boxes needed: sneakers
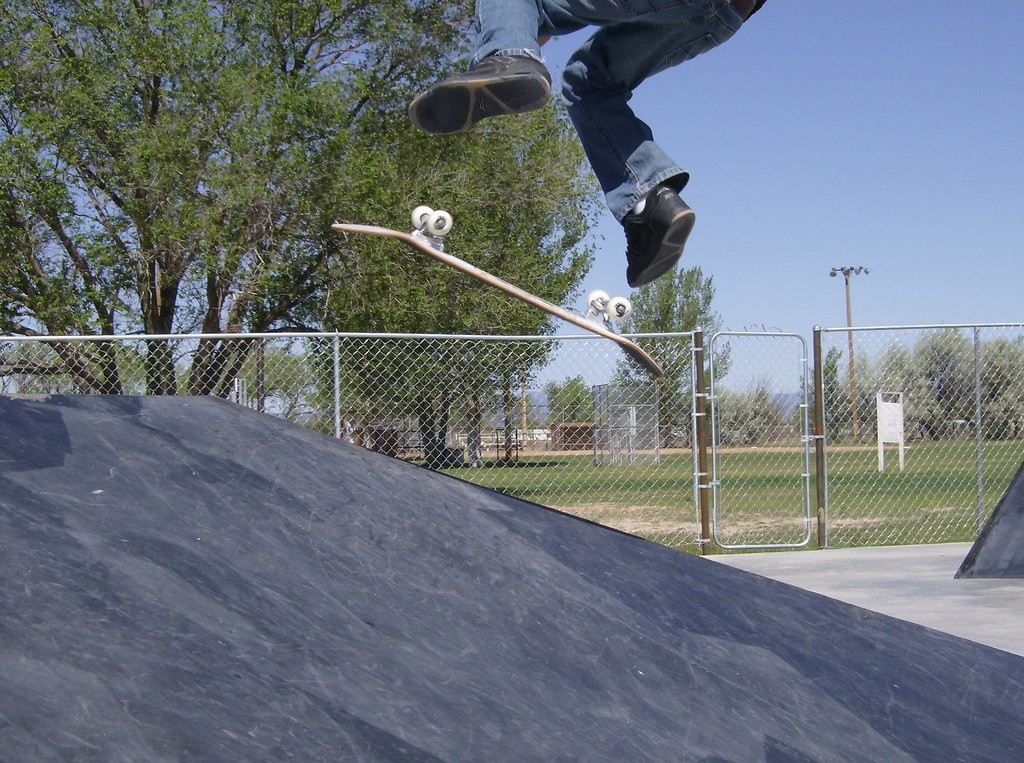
[622,185,696,287]
[408,54,552,135]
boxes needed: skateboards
[325,203,668,377]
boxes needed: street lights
[829,266,871,440]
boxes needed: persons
[408,0,767,287]
